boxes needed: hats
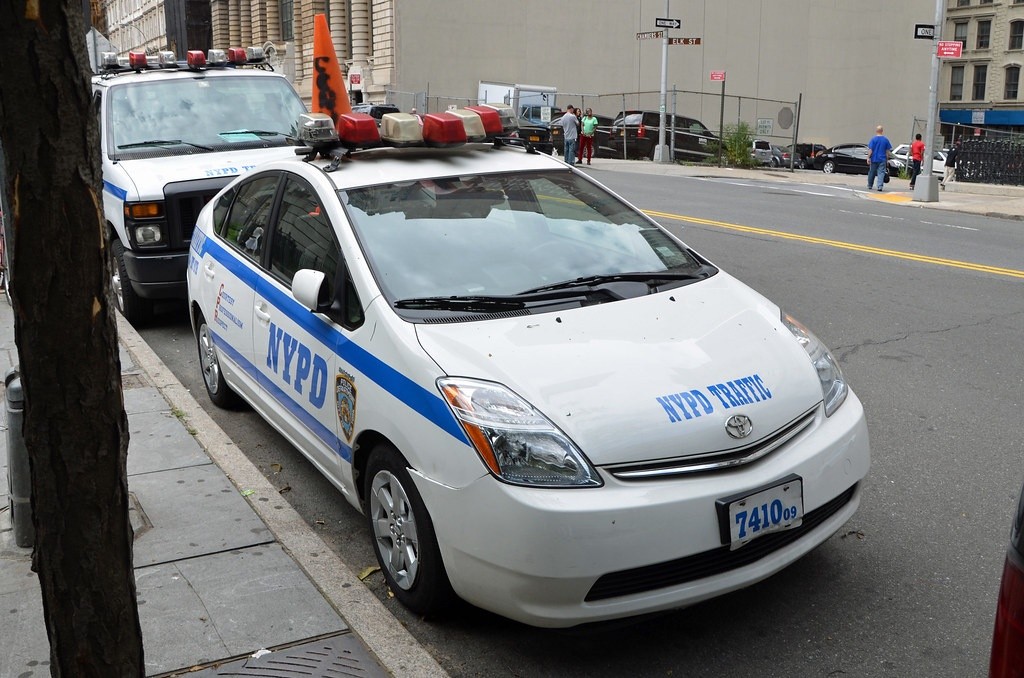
[566,104,574,109]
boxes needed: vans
[91,47,309,329]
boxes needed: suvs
[747,140,826,168]
[609,110,728,161]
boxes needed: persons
[866,124,892,191]
[561,103,599,166]
[940,141,961,190]
[909,133,926,187]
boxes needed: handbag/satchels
[884,173,889,183]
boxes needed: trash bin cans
[3,365,35,549]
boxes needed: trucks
[477,81,562,127]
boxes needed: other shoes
[576,161,582,164]
[910,185,914,190]
[877,189,882,191]
[587,161,590,165]
[940,184,945,192]
[869,187,872,189]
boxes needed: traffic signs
[655,18,681,30]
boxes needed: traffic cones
[312,13,353,135]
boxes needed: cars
[187,104,871,629]
[892,144,984,179]
[813,143,922,177]
[502,113,614,157]
